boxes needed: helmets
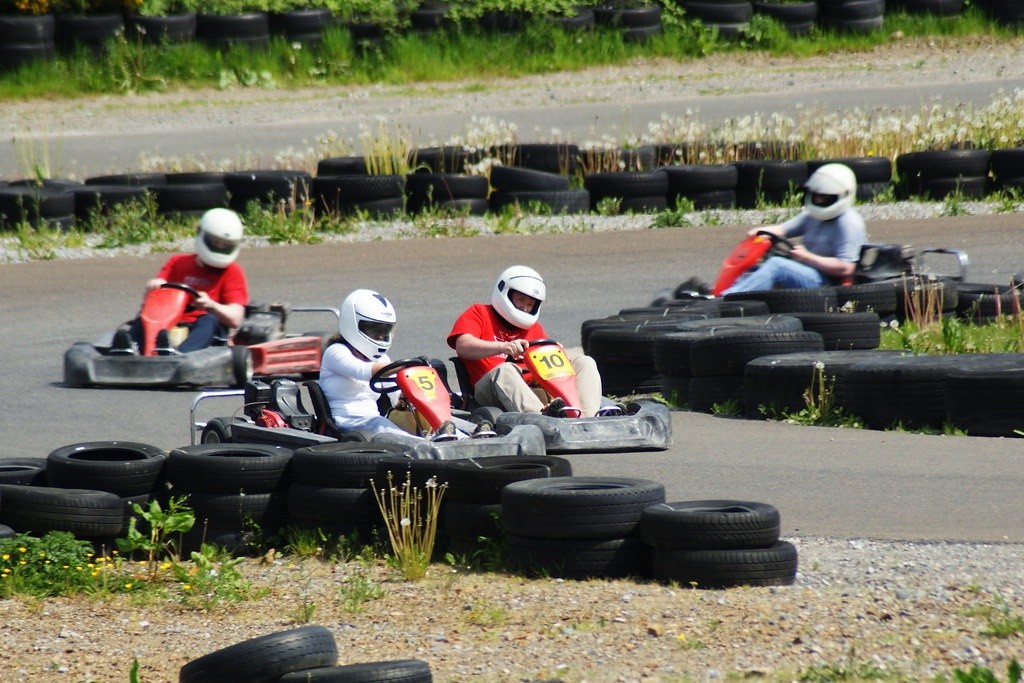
[804,163,857,221]
[195,208,244,268]
[338,289,397,362]
[491,265,547,329]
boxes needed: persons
[674,163,869,301]
[112,207,250,359]
[446,265,602,418]
[318,288,495,442]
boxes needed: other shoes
[112,325,132,348]
[155,329,171,355]
[467,419,493,440]
[540,397,566,418]
[427,419,456,441]
